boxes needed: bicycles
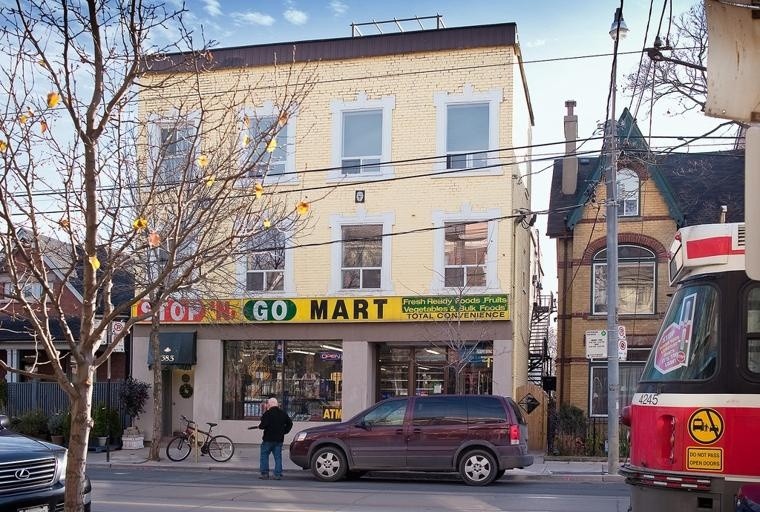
[167,414,235,461]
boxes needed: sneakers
[260,475,270,479]
[271,474,281,478]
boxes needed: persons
[257,397,294,479]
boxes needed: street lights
[599,10,631,472]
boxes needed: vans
[289,393,534,485]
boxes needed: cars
[0,414,93,512]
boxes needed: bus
[618,223,760,512]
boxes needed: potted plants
[14,399,118,449]
